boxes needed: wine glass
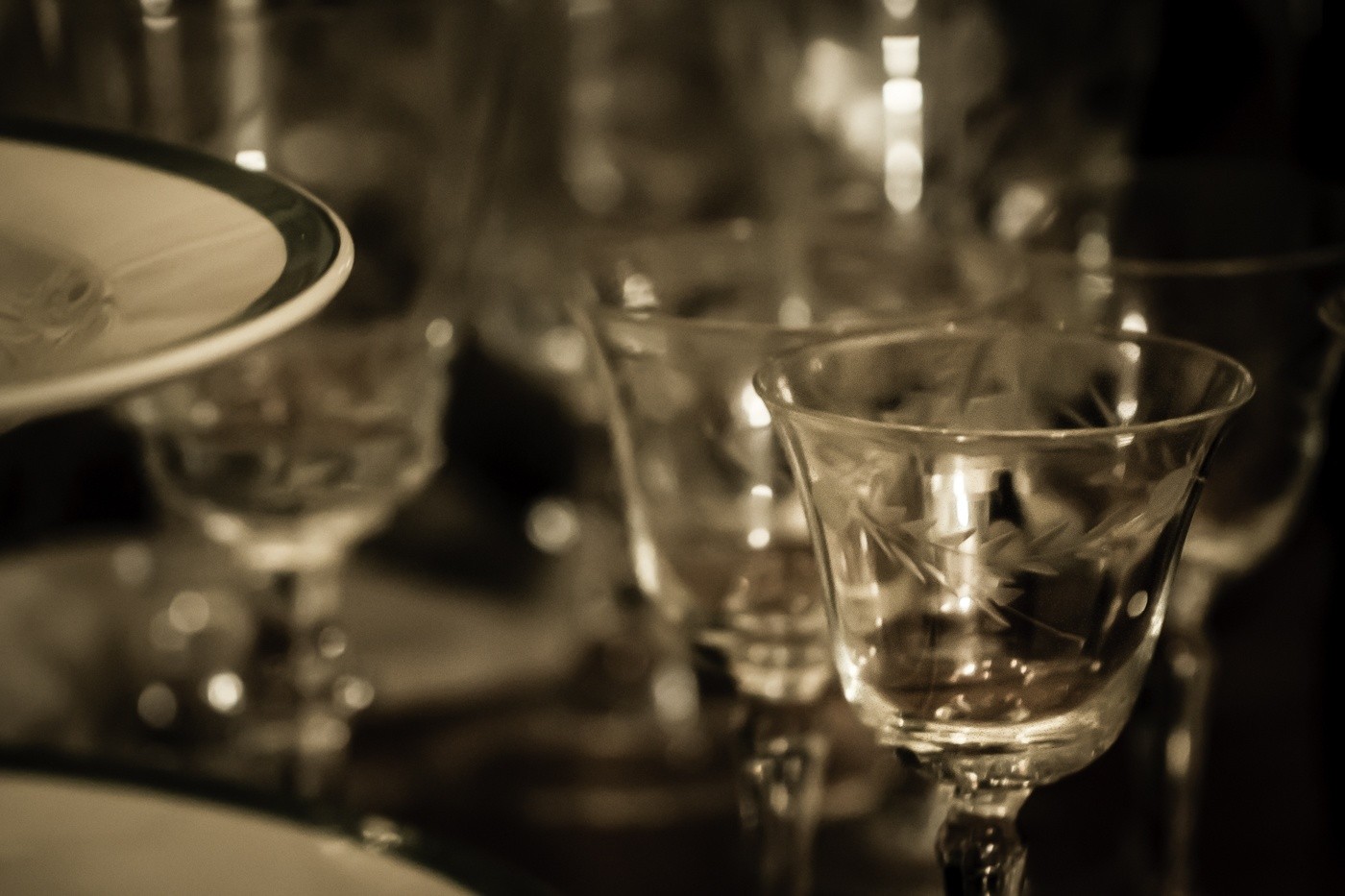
[560,164,1344,896]
[130,208,459,862]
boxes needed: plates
[0,118,357,433]
[0,746,546,896]
